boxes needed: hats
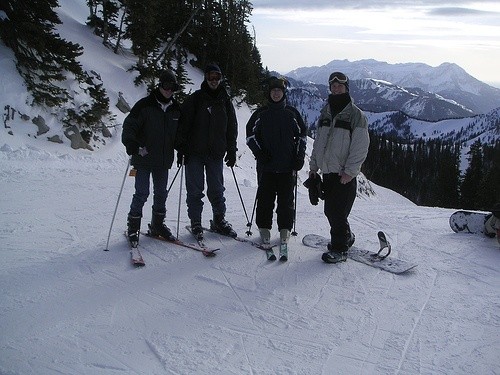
[205,64,221,72]
[269,79,286,104]
[159,69,176,83]
[329,72,350,92]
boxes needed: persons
[246,76,307,243]
[309,72,370,263]
[484,201,500,244]
[121,69,182,246]
[177,64,238,239]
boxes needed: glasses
[161,82,178,91]
[205,71,222,81]
[329,71,347,84]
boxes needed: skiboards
[122,229,221,267]
[184,224,279,257]
[259,230,288,262]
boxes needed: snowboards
[301,233,418,274]
[448,210,496,238]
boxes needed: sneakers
[191,221,203,241]
[280,229,291,245]
[210,219,237,238]
[259,228,270,244]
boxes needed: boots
[127,213,139,242]
[150,211,172,237]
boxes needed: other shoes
[322,250,347,263]
[327,234,355,252]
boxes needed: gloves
[294,155,304,171]
[303,173,326,205]
[256,150,272,162]
[225,148,237,167]
[176,144,191,165]
[130,154,147,170]
